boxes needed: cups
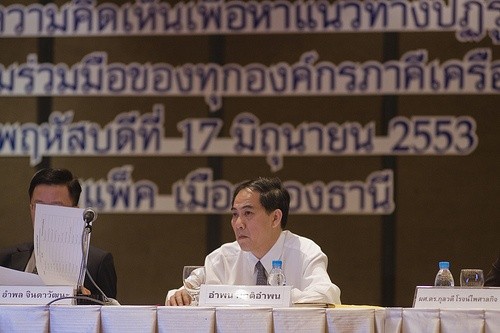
[460,269,484,286]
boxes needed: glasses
[29,203,37,210]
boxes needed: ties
[255,261,268,285]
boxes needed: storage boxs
[0,303,500,333]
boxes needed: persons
[164,177,342,307]
[483,256,500,288]
[0,167,117,305]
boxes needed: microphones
[83,207,98,224]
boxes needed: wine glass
[182,265,206,306]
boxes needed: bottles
[435,262,454,286]
[267,260,286,286]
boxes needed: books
[292,302,335,308]
